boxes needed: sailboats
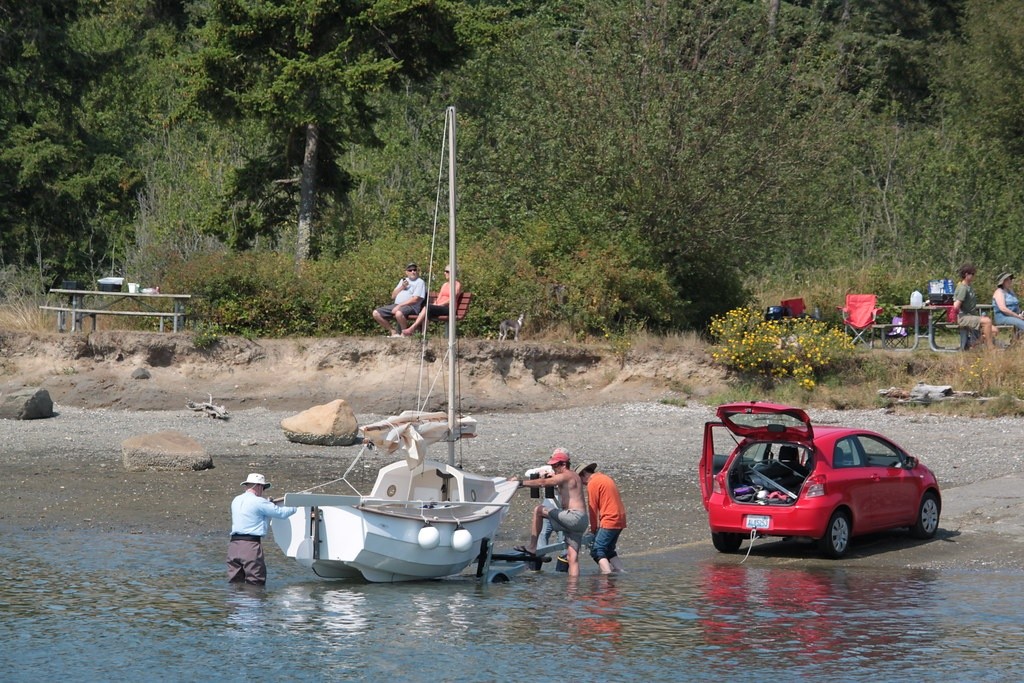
[266,108,522,584]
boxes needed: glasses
[970,272,975,275]
[405,269,417,272]
[444,270,449,273]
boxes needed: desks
[896,305,994,353]
[49,288,192,332]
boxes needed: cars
[696,402,943,559]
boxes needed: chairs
[834,446,844,466]
[835,294,882,349]
[778,446,800,464]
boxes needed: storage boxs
[98,277,124,292]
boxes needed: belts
[228,534,262,544]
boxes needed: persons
[227,474,297,586]
[993,272,1024,330]
[511,452,588,575]
[573,463,626,572]
[372,263,425,337]
[525,448,570,549]
[954,263,999,352]
[403,263,461,337]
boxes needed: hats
[404,260,418,267]
[995,272,1013,288]
[549,447,569,458]
[546,453,569,466]
[572,462,598,480]
[239,473,270,490]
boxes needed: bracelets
[519,480,523,486]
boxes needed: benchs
[387,291,471,338]
[948,324,1014,347]
[868,321,948,350]
[38,305,185,330]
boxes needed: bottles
[910,290,922,306]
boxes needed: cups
[128,283,135,293]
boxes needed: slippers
[513,545,535,556]
